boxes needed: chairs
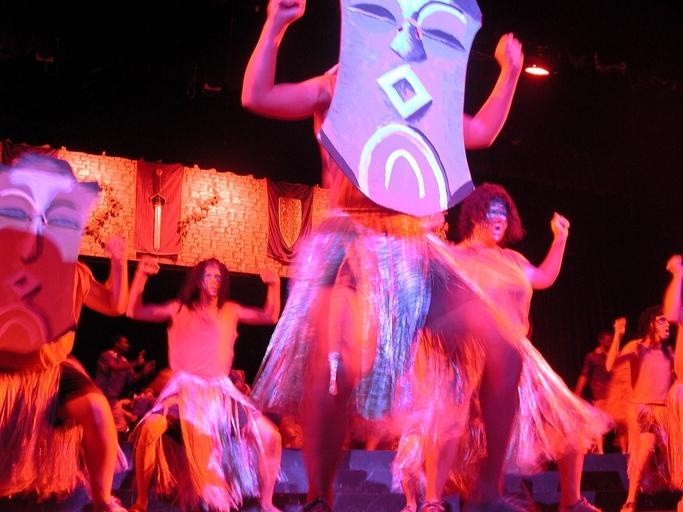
[566,495,606,512]
[501,487,542,512]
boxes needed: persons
[0,228,135,512]
[116,250,286,512]
[575,328,628,453]
[658,257,683,512]
[239,0,526,512]
[434,182,599,511]
[89,335,157,407]
[604,304,677,512]
